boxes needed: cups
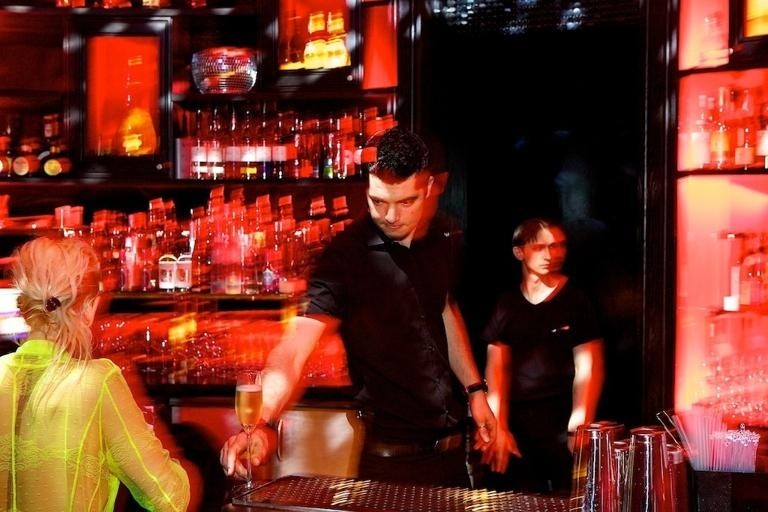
[704,349,767,429]
[425,3,594,38]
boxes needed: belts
[369,433,464,458]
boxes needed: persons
[0,234,203,512]
[482,211,607,497]
[217,127,497,490]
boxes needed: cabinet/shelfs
[644,1,768,512]
[1,1,418,427]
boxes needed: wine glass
[235,369,266,494]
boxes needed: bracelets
[465,379,490,392]
[567,430,578,438]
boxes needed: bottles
[283,11,351,74]
[697,86,767,171]
[718,230,767,311]
[1,107,73,178]
[186,107,397,182]
[88,193,352,292]
[91,296,235,371]
[567,422,689,511]
